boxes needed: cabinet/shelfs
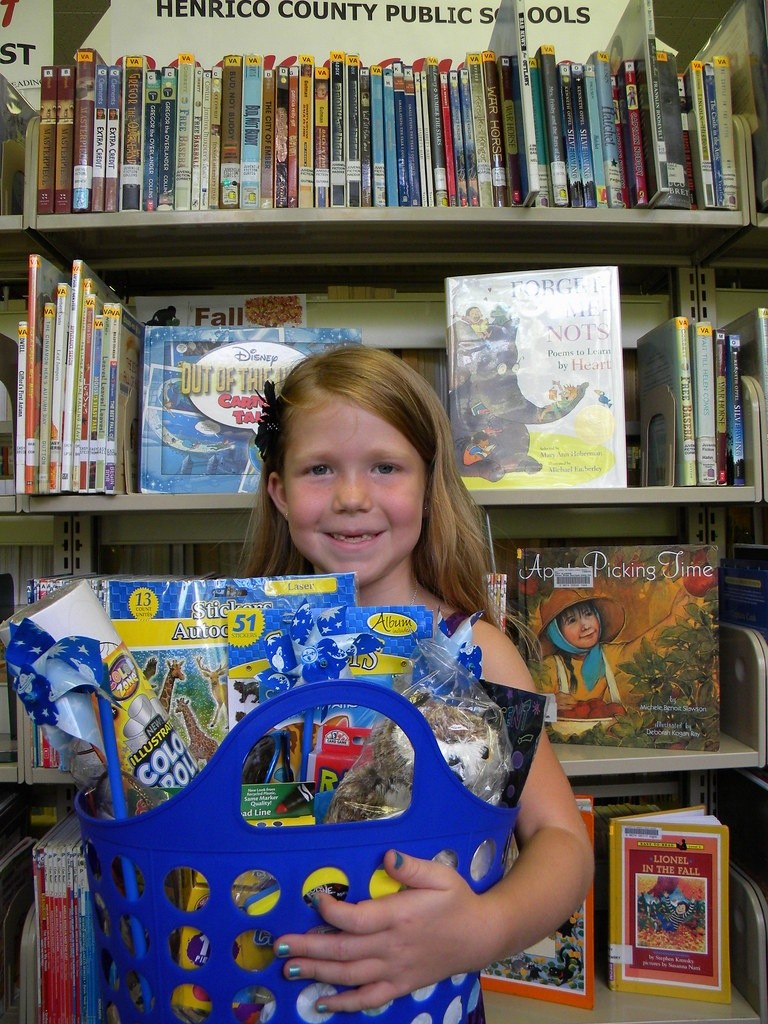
[0,0,768,1024]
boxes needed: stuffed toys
[318,686,507,828]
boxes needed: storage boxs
[137,321,365,494]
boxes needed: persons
[231,344,590,1013]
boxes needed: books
[0,37,768,1023]
